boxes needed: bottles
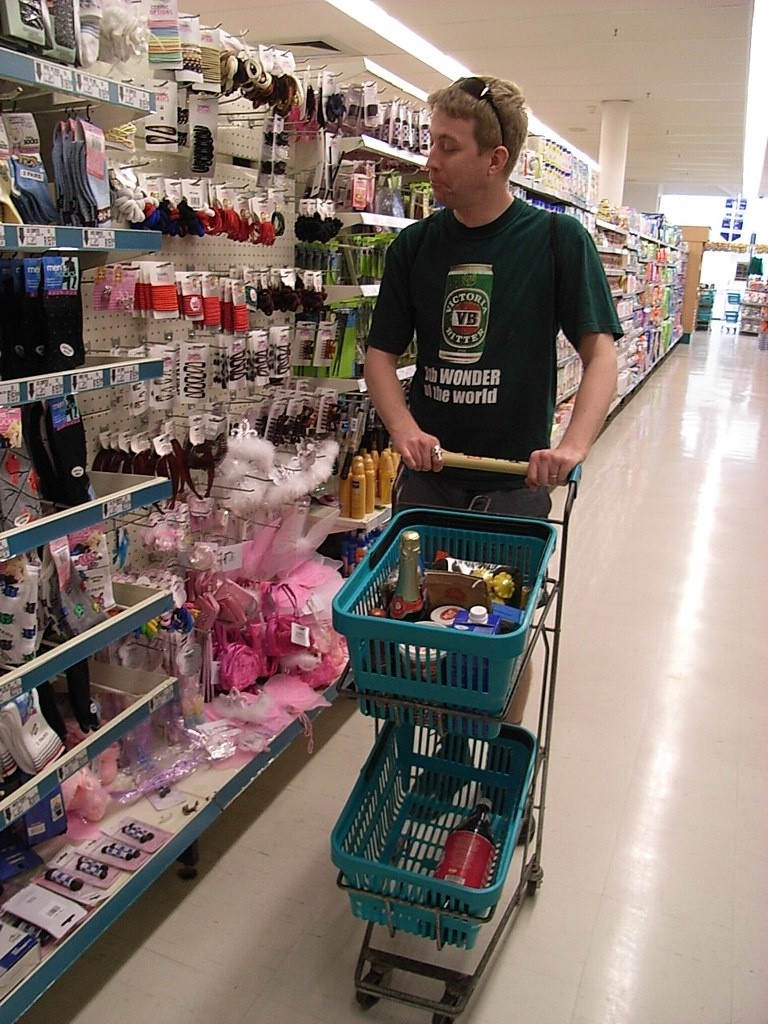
[388,531,427,682]
[420,798,495,939]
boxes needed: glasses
[450,77,506,147]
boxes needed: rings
[433,445,442,461]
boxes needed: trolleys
[719,288,742,335]
[695,283,718,333]
[329,442,584,1024]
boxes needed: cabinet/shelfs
[739,274,768,351]
[0,0,691,1024]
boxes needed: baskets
[699,293,712,306]
[330,721,537,950]
[330,508,558,741]
[725,311,738,323]
[697,310,711,322]
[728,293,740,304]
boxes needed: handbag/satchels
[185,564,346,692]
[749,256,763,275]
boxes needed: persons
[364,73,620,842]
